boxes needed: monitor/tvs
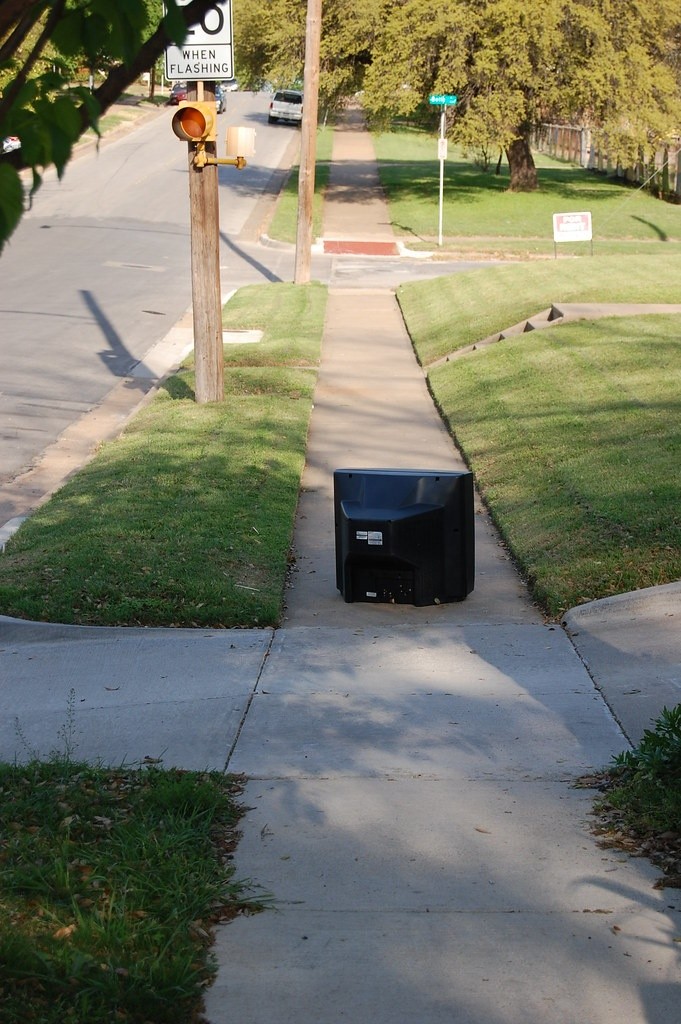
[335,468,475,607]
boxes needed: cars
[213,86,226,114]
[171,81,188,104]
[268,89,304,126]
